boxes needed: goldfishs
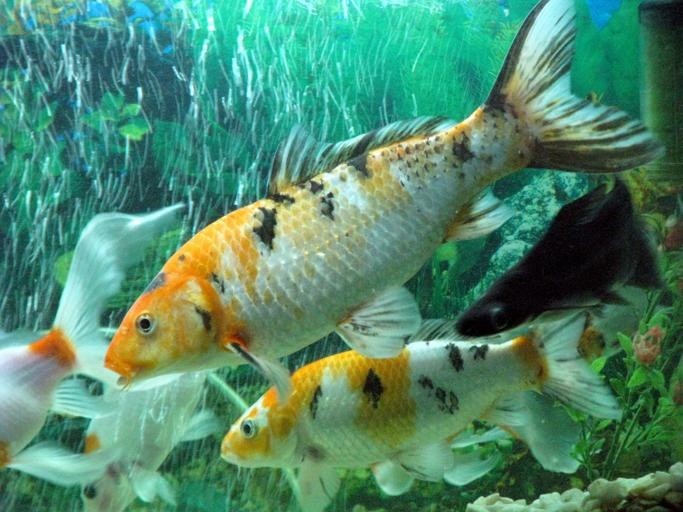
[0,201,189,488]
[80,370,228,511]
